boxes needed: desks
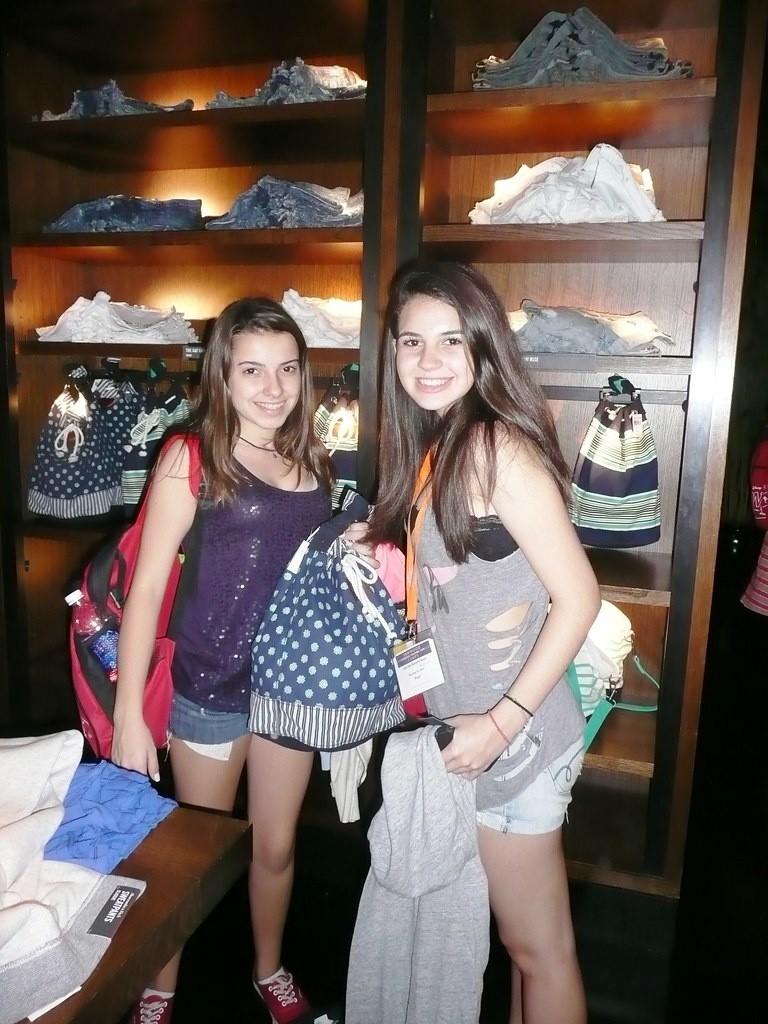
[0,805,253,1024]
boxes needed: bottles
[64,588,120,689]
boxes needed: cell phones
[434,724,500,772]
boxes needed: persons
[361,257,600,1024]
[110,296,332,1024]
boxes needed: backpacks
[70,433,202,759]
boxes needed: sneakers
[253,968,315,1024]
[128,994,175,1024]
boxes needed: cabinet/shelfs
[0,0,768,903]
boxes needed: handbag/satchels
[247,490,415,751]
[548,598,660,752]
[26,356,191,523]
[313,363,362,514]
[565,375,661,548]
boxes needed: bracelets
[487,710,512,745]
[503,693,534,717]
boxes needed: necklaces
[235,434,278,459]
